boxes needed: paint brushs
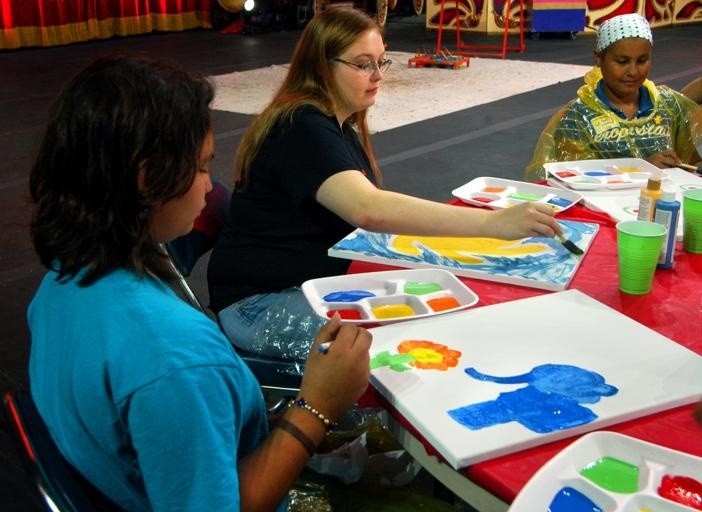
[677,164,701,173]
[557,234,583,254]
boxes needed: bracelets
[287,399,334,437]
[276,417,316,458]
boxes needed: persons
[26,50,375,512]
[207,8,562,361]
[521,11,702,180]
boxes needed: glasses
[329,55,393,75]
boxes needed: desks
[347,185,701,512]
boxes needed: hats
[596,12,654,54]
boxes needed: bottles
[638,179,662,222]
[653,192,680,269]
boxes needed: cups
[614,218,666,296]
[682,189,701,253]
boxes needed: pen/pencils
[318,340,335,352]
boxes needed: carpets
[202,52,592,135]
